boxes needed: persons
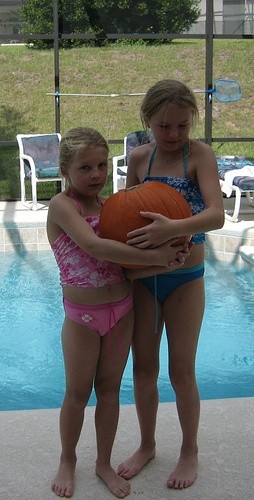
[44,123,197,500]
[117,79,226,491]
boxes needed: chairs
[214,154,254,224]
[15,132,67,213]
[111,129,155,195]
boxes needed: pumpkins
[100,180,194,269]
